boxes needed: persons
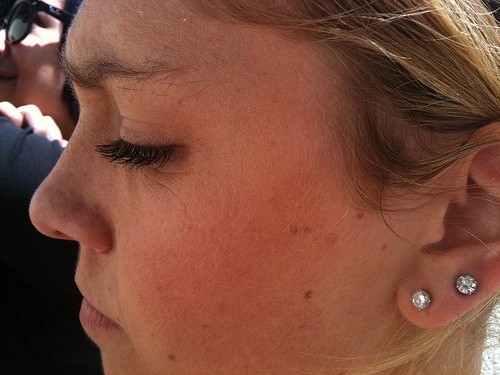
[0,0,82,147]
[29,0,500,375]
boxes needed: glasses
[0,0,74,45]
